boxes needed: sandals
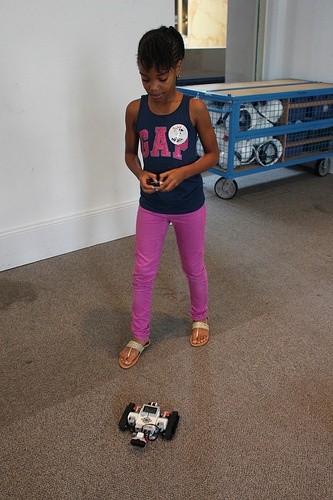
[190,316,209,346]
[118,339,150,368]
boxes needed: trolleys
[175,78,333,201]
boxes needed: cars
[118,401,180,448]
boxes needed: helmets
[189,99,285,169]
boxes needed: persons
[118,25,219,370]
